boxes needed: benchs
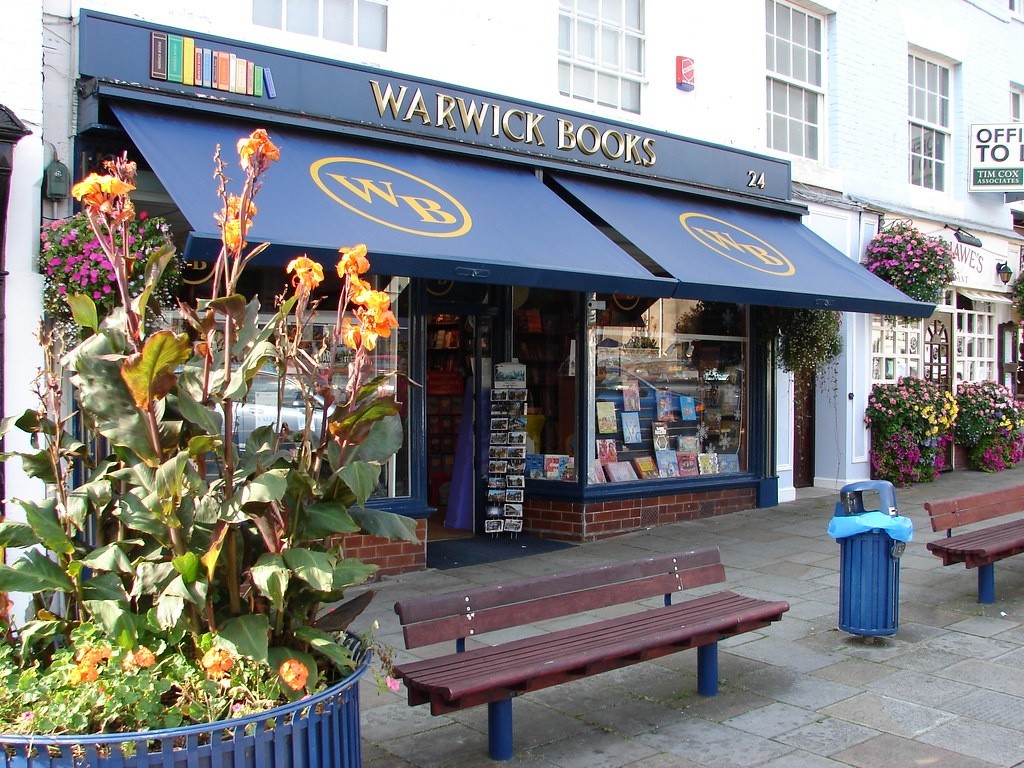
[391,547,790,761]
[924,483,1024,604]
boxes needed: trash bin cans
[836,479,907,639]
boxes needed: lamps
[944,224,982,247]
[996,261,1013,284]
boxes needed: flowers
[0,130,417,756]
[861,375,1024,487]
[862,220,958,331]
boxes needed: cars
[175,370,336,455]
[596,364,706,447]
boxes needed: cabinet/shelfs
[427,301,559,454]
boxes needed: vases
[0,630,370,768]
